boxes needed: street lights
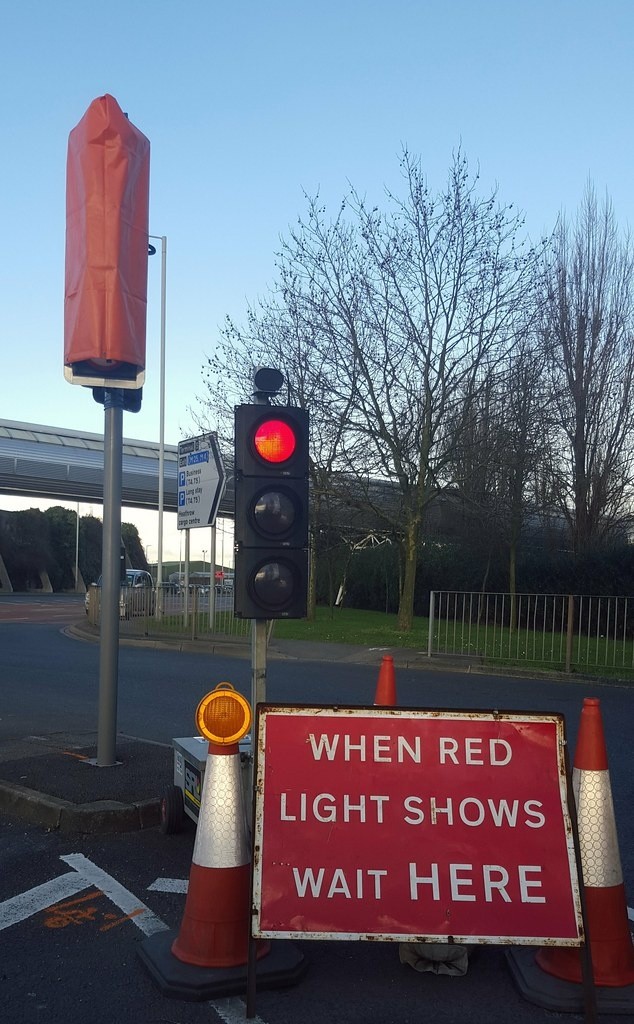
[202,550,208,573]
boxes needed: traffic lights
[232,403,314,623]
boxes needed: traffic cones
[128,687,323,1004]
[502,696,634,1016]
[376,656,399,707]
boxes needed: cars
[85,569,155,618]
[160,582,232,594]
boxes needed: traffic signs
[177,432,228,530]
